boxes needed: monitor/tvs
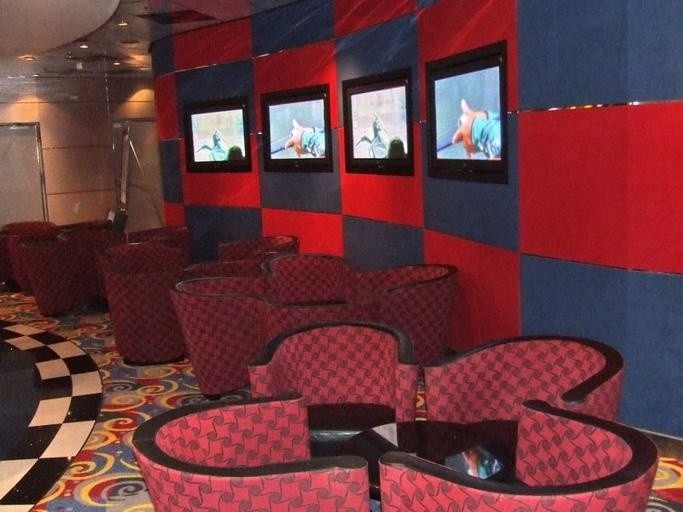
[266,92,330,165]
[346,79,413,166]
[188,104,248,165]
[430,55,506,171]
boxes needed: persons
[371,116,387,142]
[285,118,326,160]
[452,97,501,160]
[225,145,245,161]
[386,139,405,158]
[210,129,223,149]
